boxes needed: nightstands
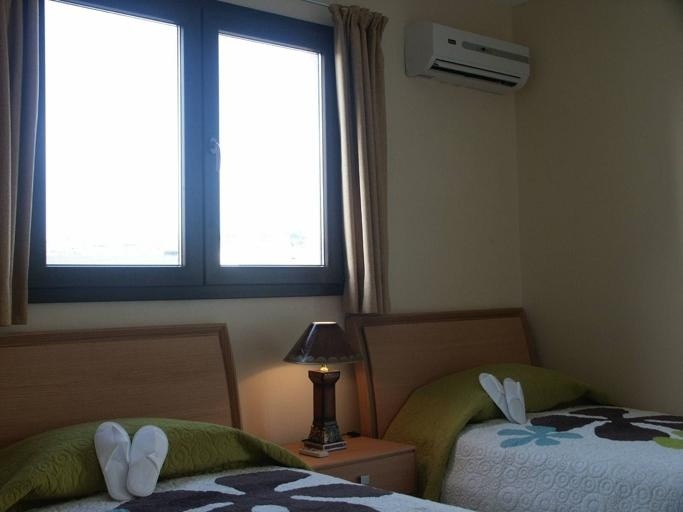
[279,433,418,495]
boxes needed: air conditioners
[404,22,530,96]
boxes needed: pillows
[436,361,574,424]
[7,418,255,507]
[444,363,582,421]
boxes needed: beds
[1,321,480,512]
[344,307,683,512]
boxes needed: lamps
[281,321,365,451]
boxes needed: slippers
[94,421,131,500]
[126,424,170,494]
[503,375,525,424]
[479,373,510,420]
